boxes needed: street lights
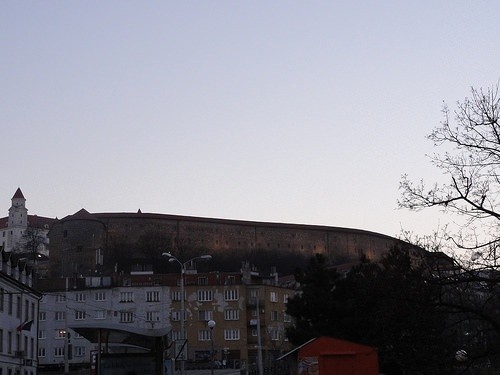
[161,251,212,375]
[208,320,216,375]
